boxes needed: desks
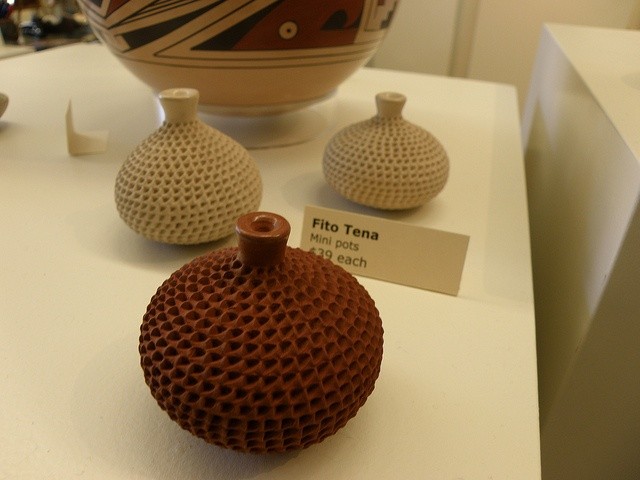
[2,44,544,480]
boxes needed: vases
[323,92,448,206]
[138,211,384,455]
[78,1,398,151]
[114,87,263,245]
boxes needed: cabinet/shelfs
[521,22,636,472]
